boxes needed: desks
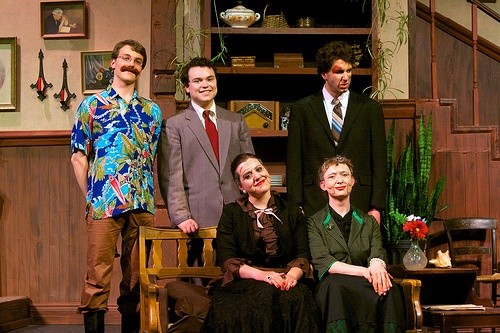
[388,267,479,305]
[421,304,500,333]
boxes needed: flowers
[388,207,428,244]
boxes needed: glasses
[119,55,143,66]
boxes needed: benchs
[139,225,219,333]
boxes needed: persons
[285,41,387,224]
[306,155,405,333]
[70,41,162,333]
[156,57,256,287]
[201,152,319,333]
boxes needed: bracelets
[369,257,386,269]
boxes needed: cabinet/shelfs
[200,0,379,194]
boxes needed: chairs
[443,217,500,307]
[392,277,423,333]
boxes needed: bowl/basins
[220,5,261,29]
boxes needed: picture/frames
[80,51,114,95]
[40,0,88,40]
[0,36,18,112]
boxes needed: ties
[204,109,219,162]
[331,99,344,143]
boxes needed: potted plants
[383,109,452,264]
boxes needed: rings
[268,276,271,280]
[281,276,285,279]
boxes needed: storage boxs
[230,56,256,67]
[230,99,280,131]
[273,53,304,69]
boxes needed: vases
[402,243,427,271]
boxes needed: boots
[83,311,105,333]
[121,313,140,333]
